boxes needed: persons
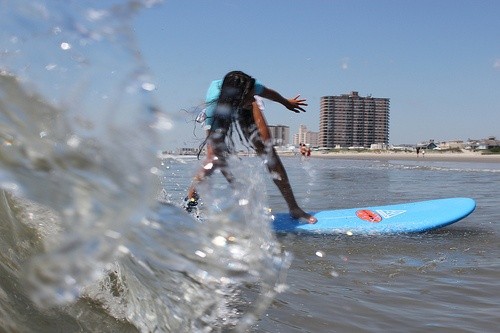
[185,70,319,224]
[416,144,421,159]
[299,143,311,157]
[422,148,425,158]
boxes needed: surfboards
[266,196,476,236]
